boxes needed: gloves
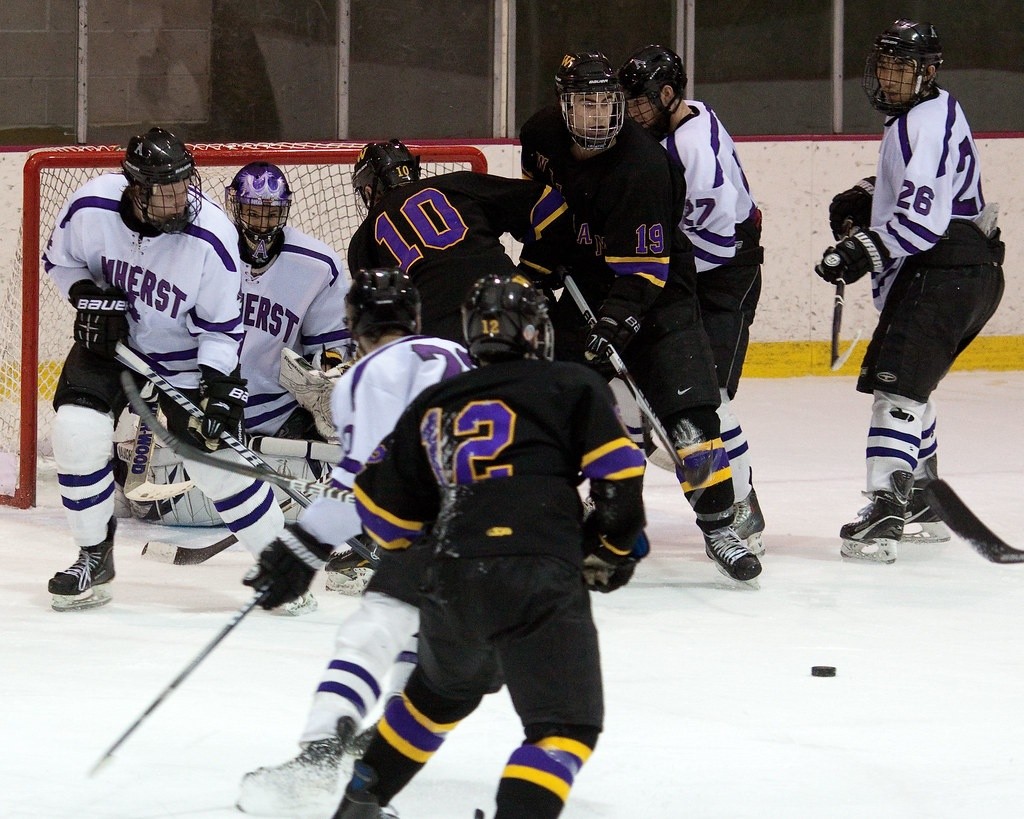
[815,232,887,286]
[68,279,132,361]
[188,374,248,453]
[244,523,334,610]
[582,528,635,592]
[579,298,641,380]
[828,176,877,240]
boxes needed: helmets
[352,139,420,222]
[121,126,201,233]
[863,20,942,114]
[225,162,292,246]
[556,51,625,150]
[618,44,688,131]
[343,266,423,339]
[459,271,557,364]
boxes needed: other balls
[812,665,836,677]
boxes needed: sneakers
[326,539,379,594]
[333,762,399,819]
[254,581,317,616]
[48,517,117,611]
[696,518,762,590]
[240,740,344,805]
[839,470,915,564]
[863,453,951,543]
[583,495,597,523]
[706,466,765,562]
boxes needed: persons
[236,269,482,819]
[328,277,649,819]
[816,19,1005,561]
[48,43,769,615]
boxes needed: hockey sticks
[121,370,356,505]
[139,471,332,567]
[114,341,382,570]
[920,478,1024,564]
[87,583,270,776]
[828,219,863,373]
[122,403,195,502]
[564,273,717,486]
